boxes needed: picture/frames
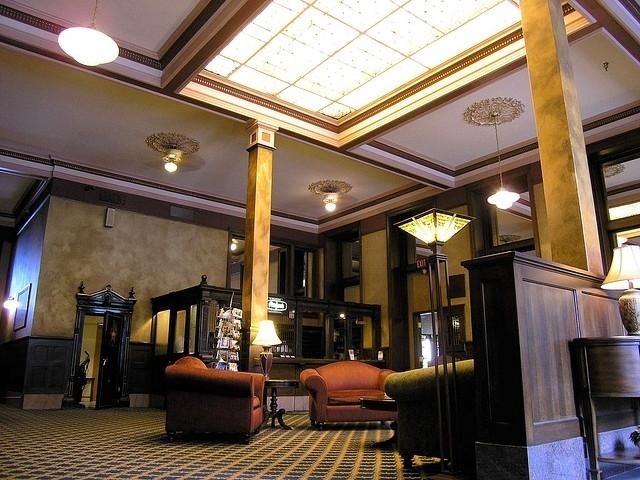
[13,283,31,330]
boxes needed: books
[216,308,243,371]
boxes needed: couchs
[301,360,397,425]
[384,360,474,468]
[164,354,266,437]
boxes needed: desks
[571,335,640,480]
[262,377,299,430]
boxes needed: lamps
[4,298,17,310]
[485,119,521,211]
[601,245,640,335]
[55,1,119,67]
[321,191,339,211]
[160,146,183,174]
[252,322,283,380]
[394,207,476,480]
